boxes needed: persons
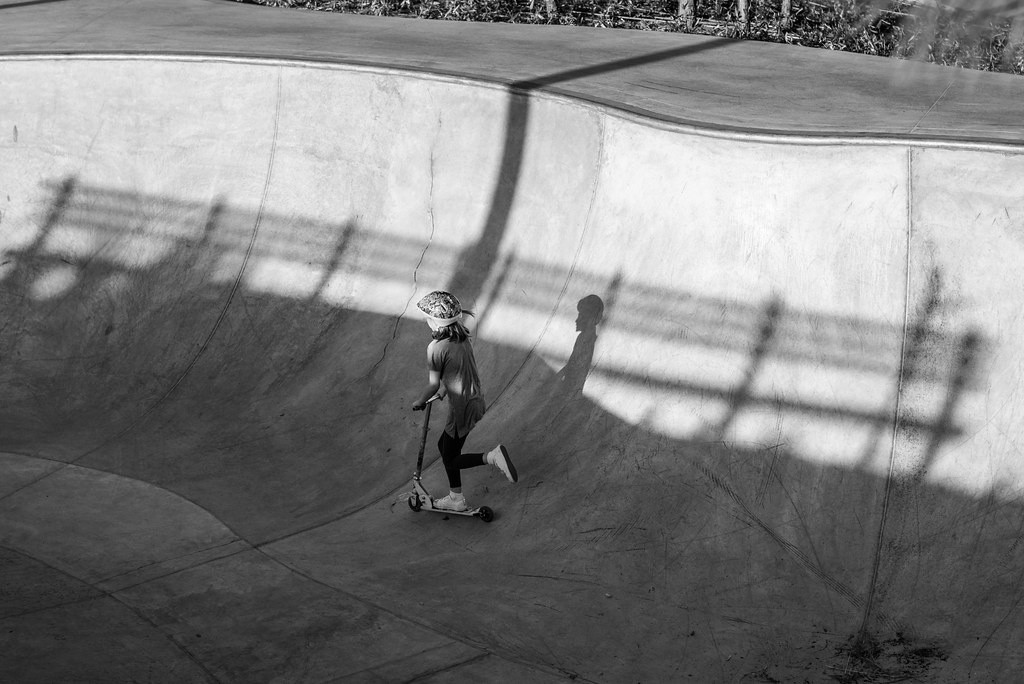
[413,291,518,512]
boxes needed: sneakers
[433,495,467,511]
[487,445,517,483]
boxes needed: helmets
[417,291,462,320]
